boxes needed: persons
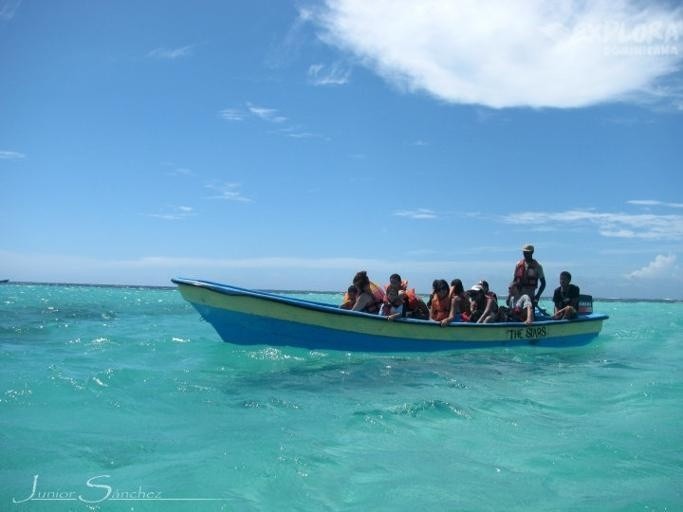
[552,271,580,319]
[505,243,546,307]
[340,271,534,328]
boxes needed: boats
[168,274,609,354]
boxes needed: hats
[467,285,484,292]
[522,244,534,251]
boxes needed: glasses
[437,285,446,292]
